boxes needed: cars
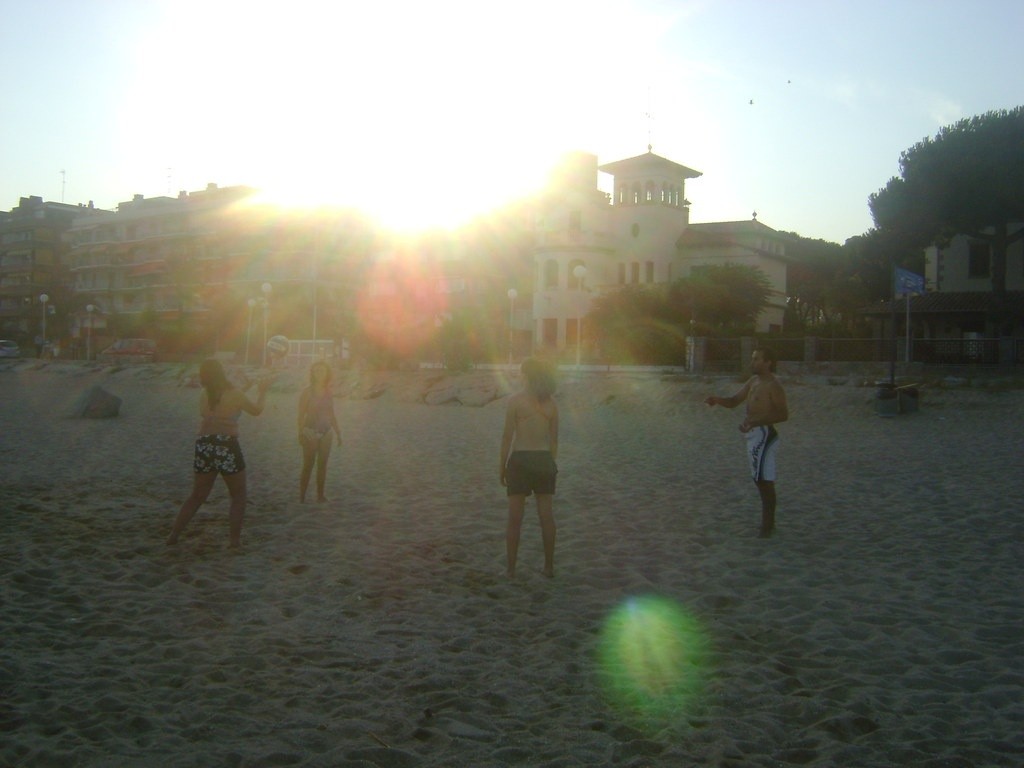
[0,339,21,359]
[100,338,160,366]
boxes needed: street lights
[573,264,587,369]
[40,293,48,356]
[260,280,274,368]
[505,288,518,367]
[85,303,94,360]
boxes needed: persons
[704,347,790,536]
[166,358,272,548]
[33,332,43,358]
[296,361,341,504]
[499,359,559,579]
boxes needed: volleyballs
[267,335,290,357]
